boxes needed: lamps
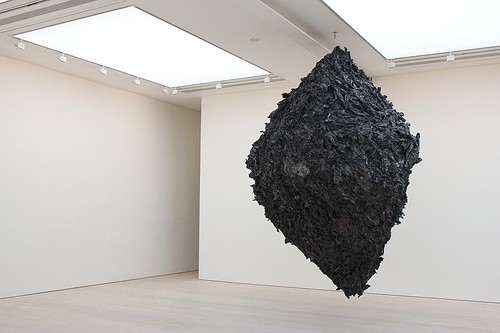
[216,80,222,89]
[161,85,168,94]
[132,75,141,85]
[172,86,179,95]
[387,58,395,69]
[263,74,271,83]
[15,39,25,50]
[58,51,66,63]
[99,64,106,74]
[446,51,454,61]
